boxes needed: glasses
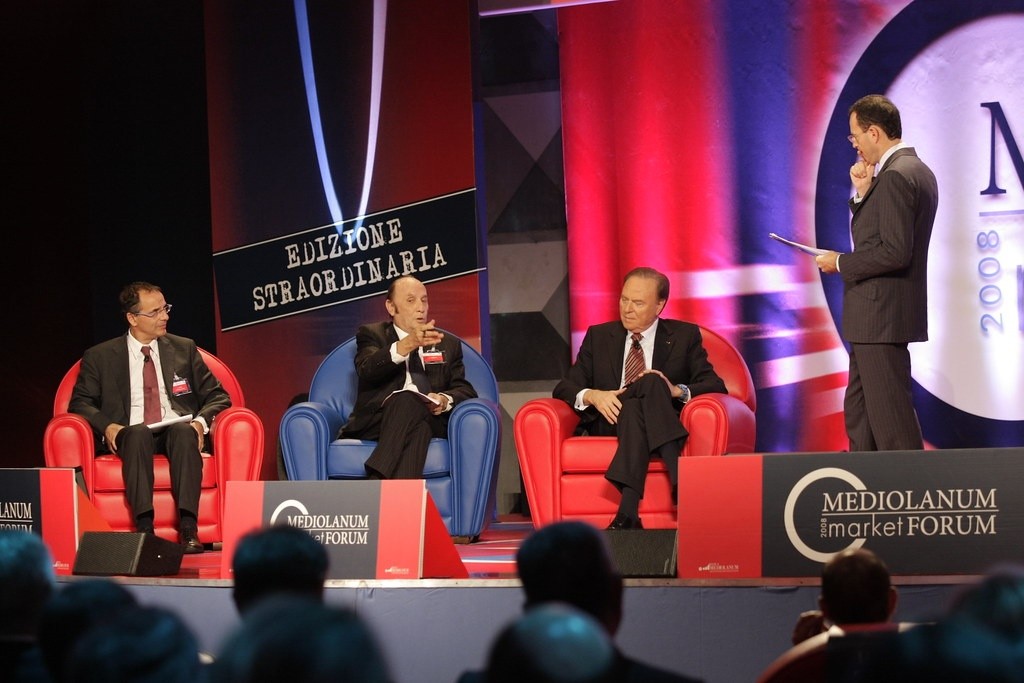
[847,124,874,143]
[133,303,172,320]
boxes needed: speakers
[599,521,678,579]
[71,531,185,577]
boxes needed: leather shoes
[179,525,203,553]
[603,512,644,531]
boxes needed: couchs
[42,346,265,549]
[514,327,757,545]
[281,325,503,544]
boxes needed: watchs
[676,383,688,400]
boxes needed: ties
[408,345,432,395]
[625,334,646,386]
[141,346,162,425]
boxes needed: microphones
[634,339,639,348]
[144,355,149,362]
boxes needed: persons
[335,277,479,480]
[0,524,390,683]
[552,267,729,529]
[67,282,232,552]
[928,568,1024,683]
[457,521,703,683]
[816,95,939,451]
[792,549,898,644]
